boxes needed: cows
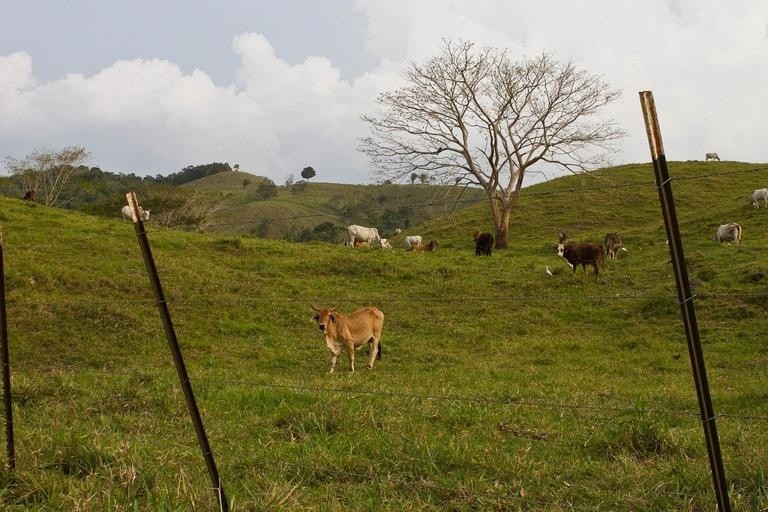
[343,224,440,253]
[749,187,768,210]
[716,220,743,244]
[705,152,721,161]
[551,231,624,278]
[307,300,387,375]
[471,227,495,258]
